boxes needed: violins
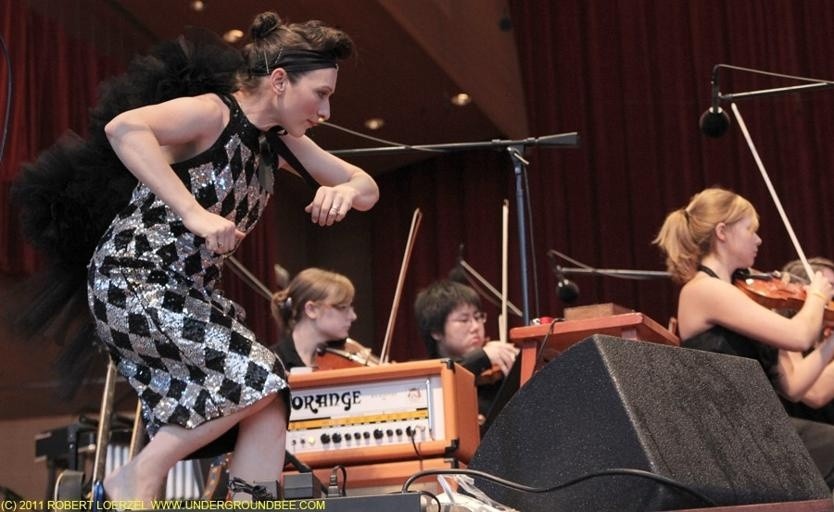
[471,335,506,388]
[311,336,396,371]
[733,266,834,333]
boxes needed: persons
[414,279,520,425]
[85,9,381,511]
[651,187,834,493]
[782,257,834,426]
[268,267,358,378]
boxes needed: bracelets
[809,288,828,300]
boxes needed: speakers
[457,333,832,512]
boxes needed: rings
[217,241,223,247]
[331,208,339,214]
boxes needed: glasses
[446,311,488,326]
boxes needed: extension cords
[234,493,421,512]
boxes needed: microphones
[699,65,730,139]
[547,255,580,303]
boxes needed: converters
[253,480,281,502]
[284,472,321,500]
